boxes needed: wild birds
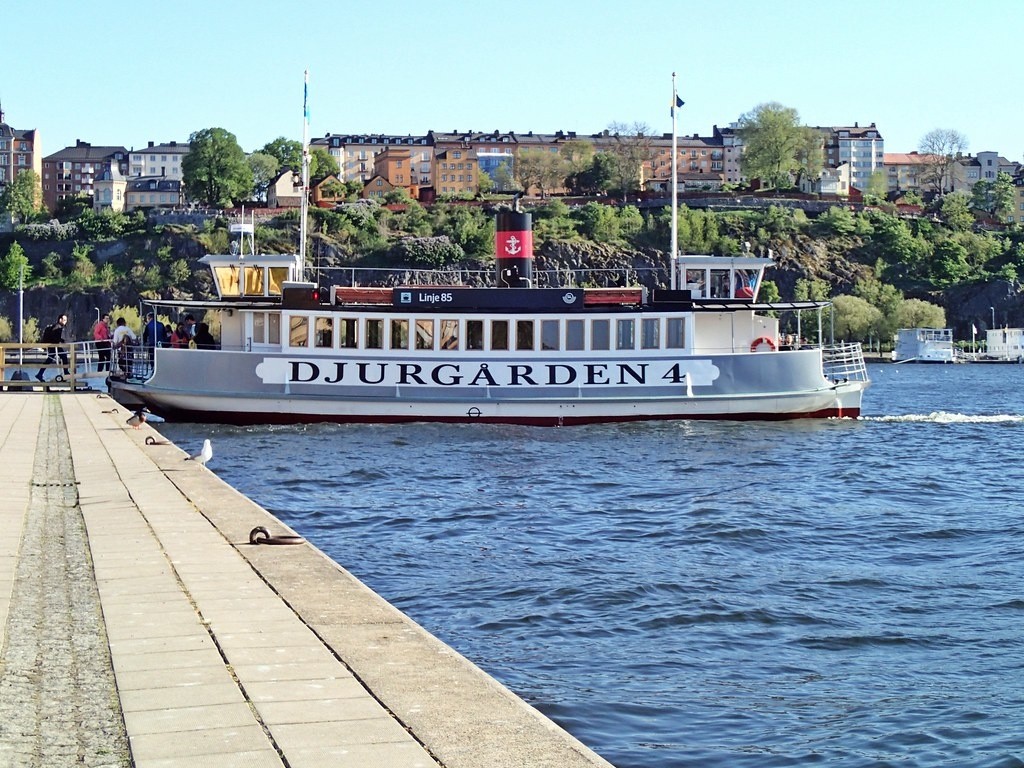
[185,439,213,467]
[126,406,151,430]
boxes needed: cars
[27,348,44,354]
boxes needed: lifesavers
[748,336,780,354]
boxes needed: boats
[103,70,853,427]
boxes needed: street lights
[95,307,99,323]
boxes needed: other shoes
[35,374,45,382]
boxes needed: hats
[799,336,808,342]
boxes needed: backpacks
[42,324,52,349]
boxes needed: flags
[670,87,685,117]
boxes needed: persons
[35,313,70,383]
[94,311,216,379]
[778,334,813,351]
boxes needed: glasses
[179,326,184,330]
